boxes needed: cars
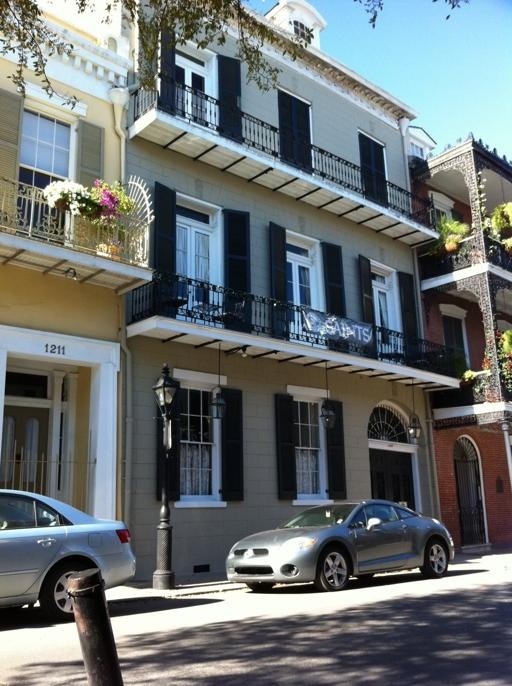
[1,489,138,621]
[225,500,454,591]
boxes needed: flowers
[90,175,135,253]
[42,179,92,216]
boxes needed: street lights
[151,363,183,595]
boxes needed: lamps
[406,377,422,438]
[208,341,228,418]
[241,346,247,358]
[320,359,336,430]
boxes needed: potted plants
[431,213,472,253]
